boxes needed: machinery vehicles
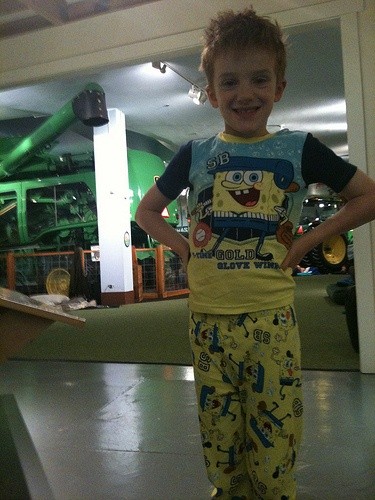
[1,80,182,303]
[291,196,357,274]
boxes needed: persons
[135,9,375,500]
